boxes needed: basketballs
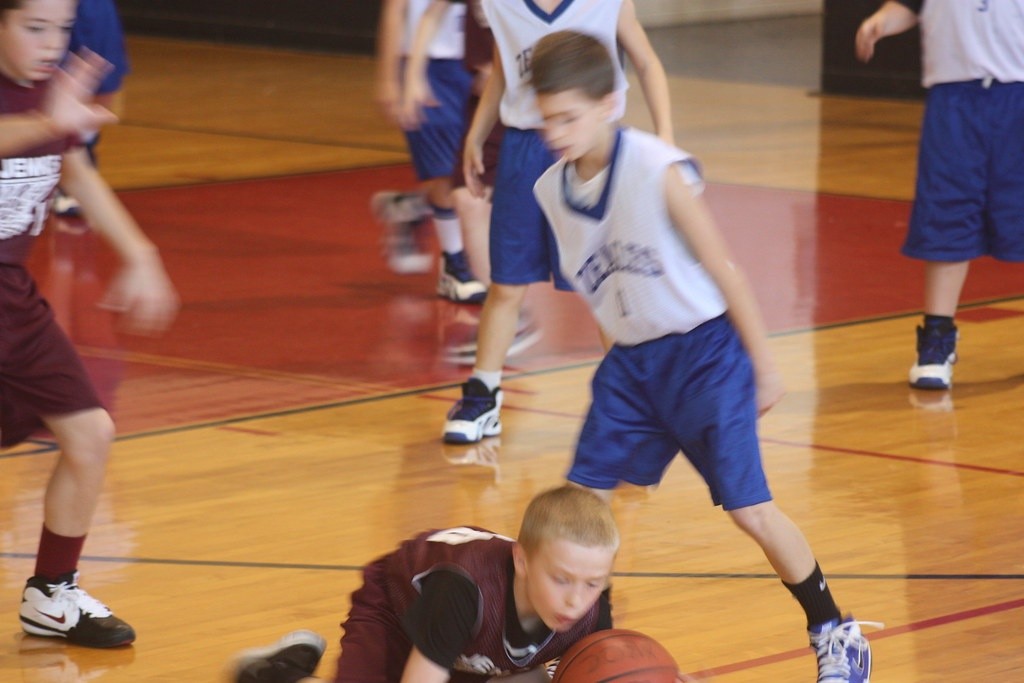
[551,629,679,683]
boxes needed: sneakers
[910,318,959,389]
[19,570,135,647]
[228,630,327,683]
[371,191,434,274]
[436,251,488,303]
[807,612,871,683]
[442,378,504,441]
[446,313,541,364]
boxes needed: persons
[440,0,676,444]
[233,483,688,683]
[531,30,871,683]
[376,0,489,306]
[1,0,181,649]
[858,1,1024,392]
[54,0,129,216]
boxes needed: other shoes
[52,185,79,215]
